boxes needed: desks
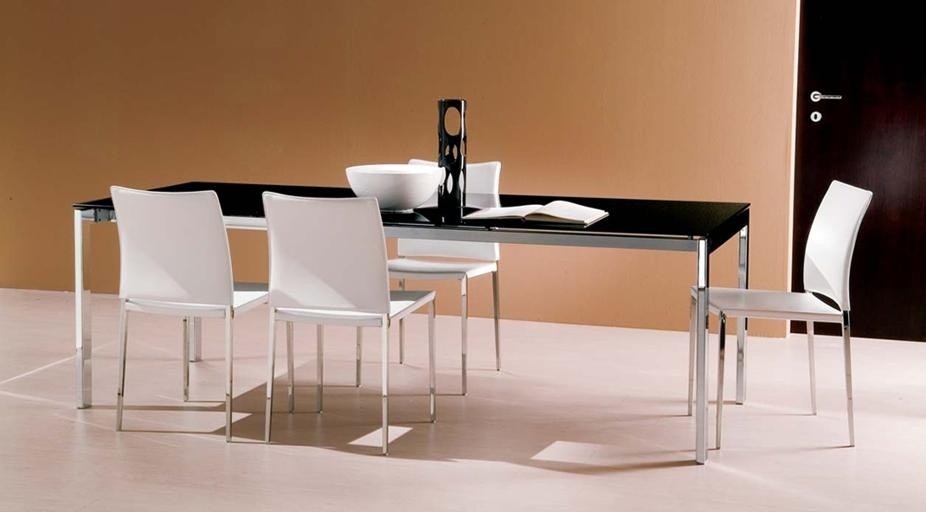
[72,182,751,466]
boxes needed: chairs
[353,158,503,396]
[262,191,437,456]
[688,179,874,449]
[110,185,294,444]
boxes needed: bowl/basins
[345,165,444,215]
